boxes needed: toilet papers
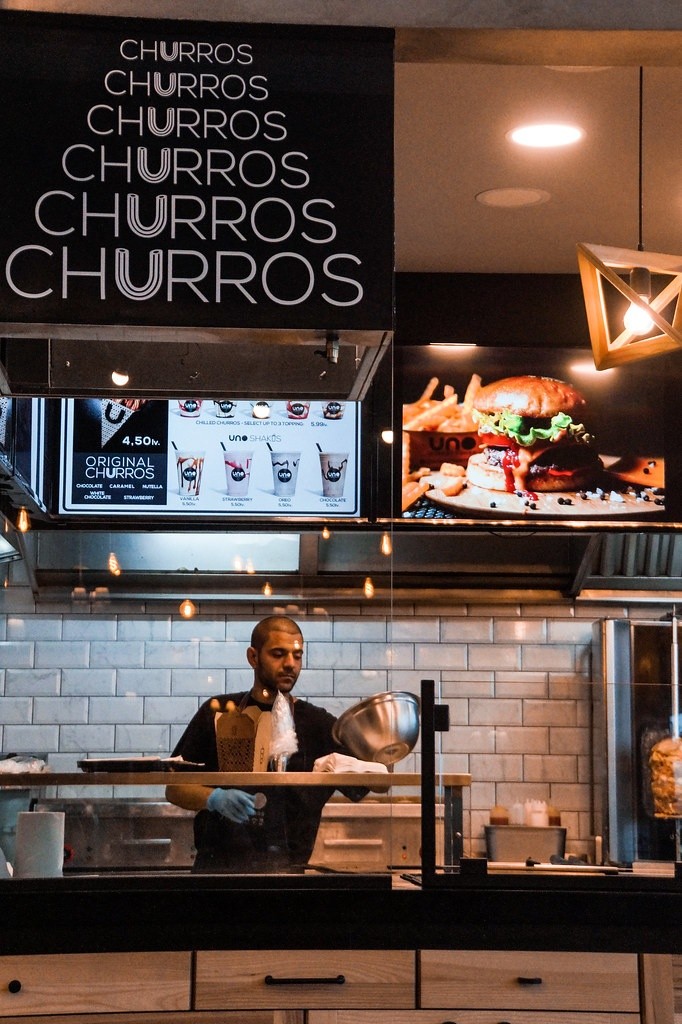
[13,812,64,877]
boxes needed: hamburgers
[465,375,605,493]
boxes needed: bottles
[490,800,509,825]
[529,801,548,827]
[509,798,524,824]
[547,806,561,827]
[524,798,540,825]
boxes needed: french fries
[402,373,484,431]
[402,461,465,511]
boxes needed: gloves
[207,787,258,825]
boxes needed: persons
[164,614,394,873]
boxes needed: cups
[402,402,485,482]
[319,452,349,497]
[174,450,206,496]
[285,401,310,419]
[213,400,237,418]
[321,402,346,420]
[270,451,301,496]
[223,451,254,496]
[250,401,274,418]
[177,400,203,417]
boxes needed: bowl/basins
[333,691,421,764]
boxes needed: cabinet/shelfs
[0,890,682,1024]
